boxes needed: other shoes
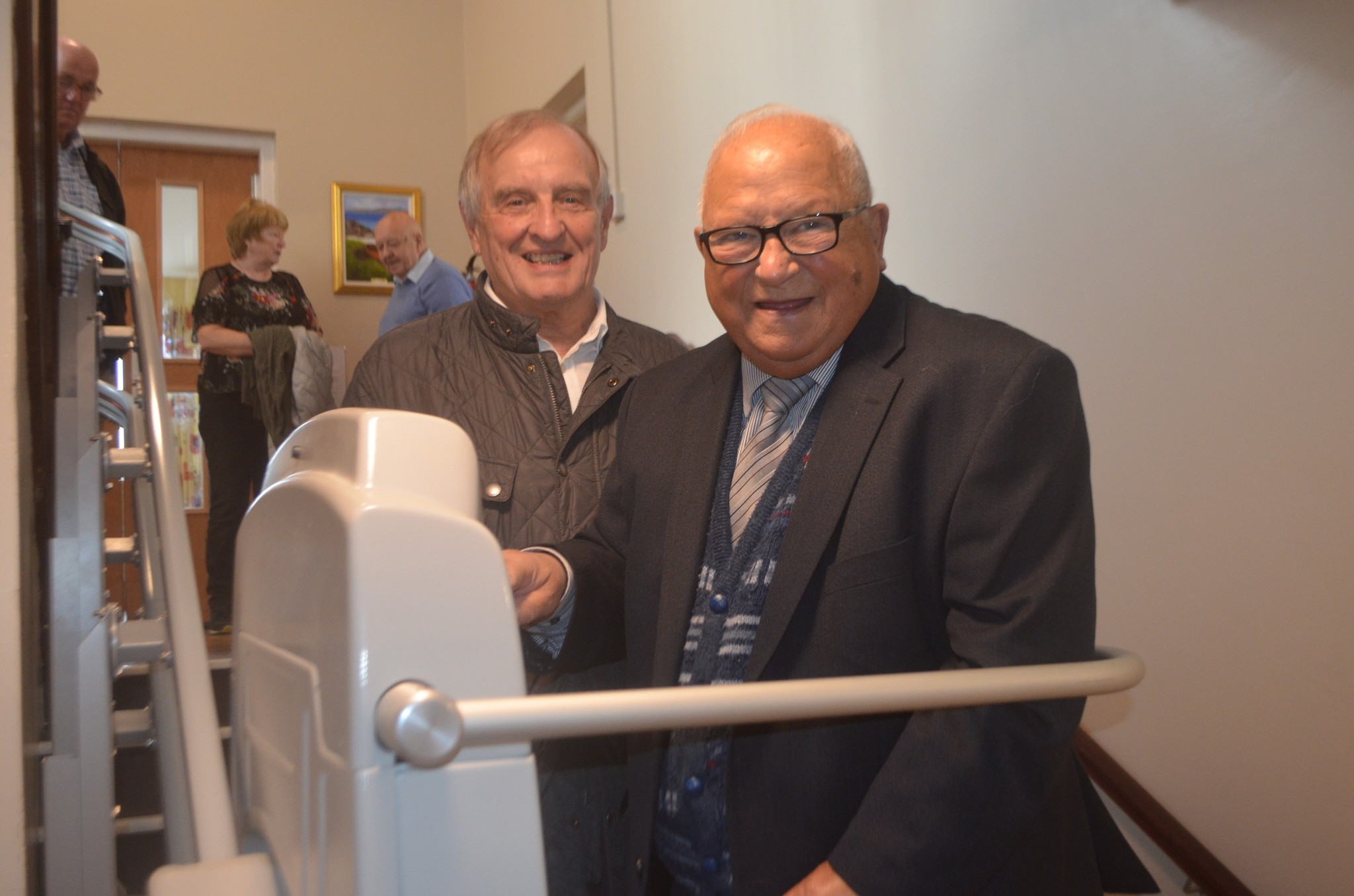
[208,616,232,634]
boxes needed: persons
[373,211,475,340]
[341,109,691,696]
[189,200,323,632]
[54,36,127,370]
[499,101,1096,896]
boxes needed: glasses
[58,75,103,103]
[700,200,870,267]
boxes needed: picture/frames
[332,182,423,296]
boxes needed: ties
[728,370,817,557]
